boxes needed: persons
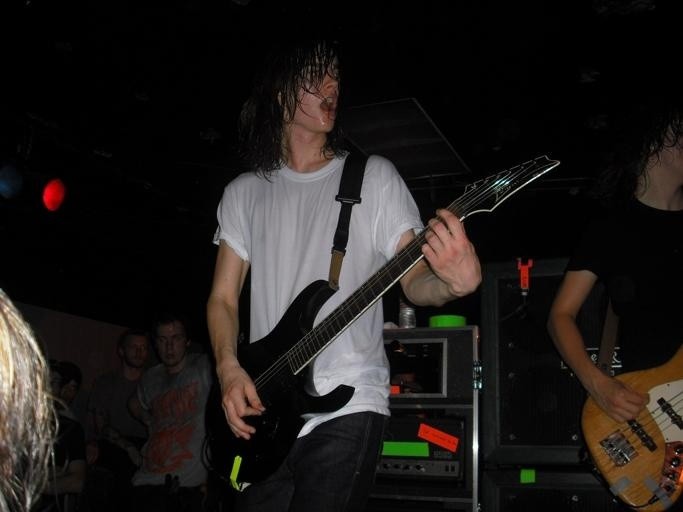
[81,328,157,464]
[0,286,60,512]
[204,34,486,512]
[42,357,87,495]
[546,107,682,424]
[126,313,217,488]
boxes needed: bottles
[399,300,415,327]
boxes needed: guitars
[580,347,683,512]
[202,156,560,488]
